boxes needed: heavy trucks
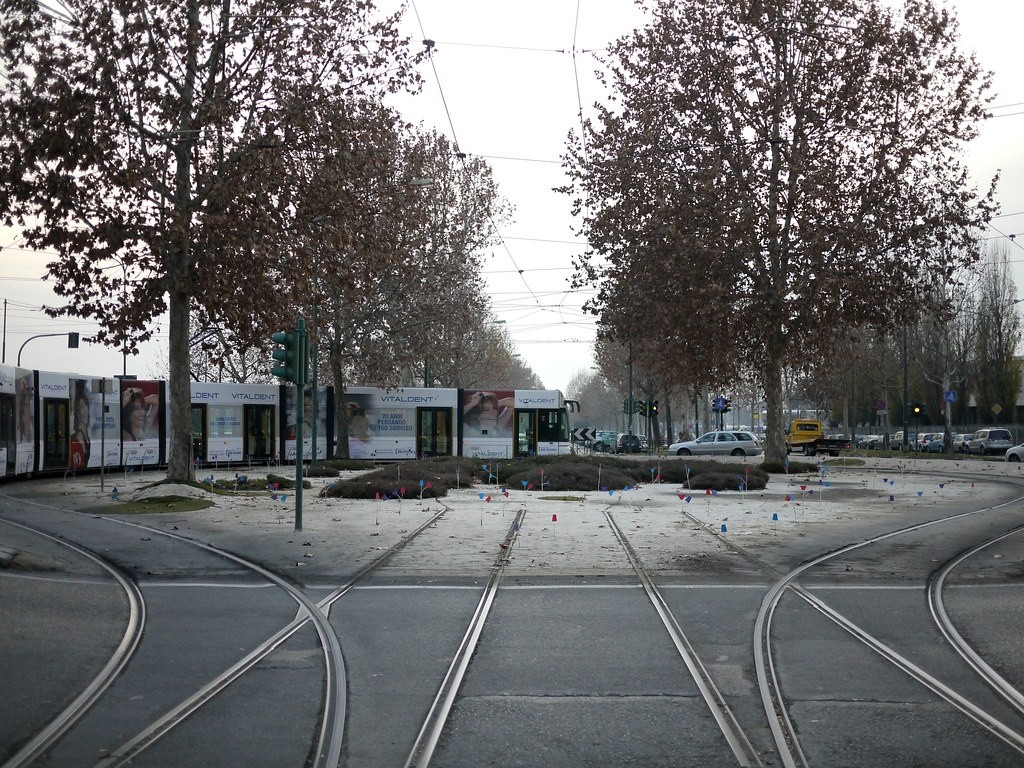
[784,418,852,457]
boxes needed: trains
[0,363,580,485]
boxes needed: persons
[303,389,320,436]
[346,402,375,450]
[71,390,91,468]
[463,392,515,437]
[19,378,34,442]
[122,388,159,441]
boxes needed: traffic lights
[712,396,731,413]
[621,399,658,417]
[915,404,921,417]
[271,328,300,386]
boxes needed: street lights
[311,178,434,466]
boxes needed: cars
[576,430,649,454]
[825,427,1024,462]
[666,429,762,456]
